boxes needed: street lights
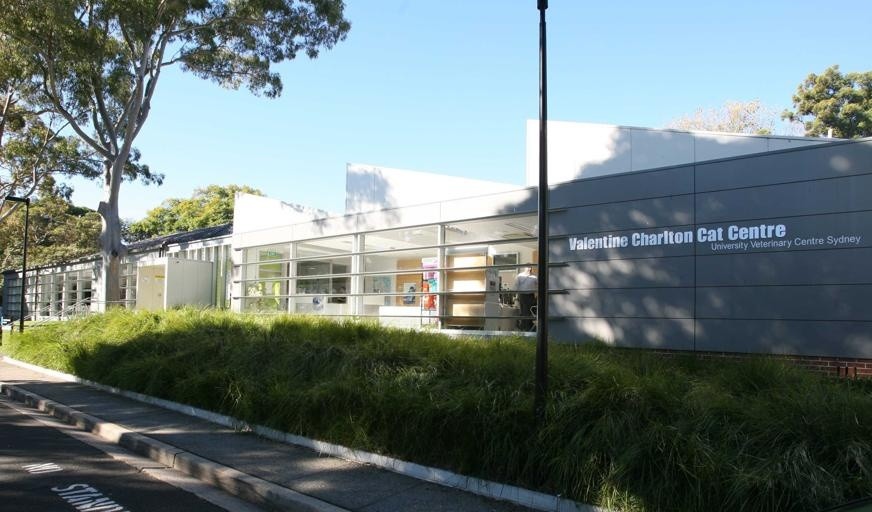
[5,196,30,333]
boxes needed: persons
[510,263,538,333]
